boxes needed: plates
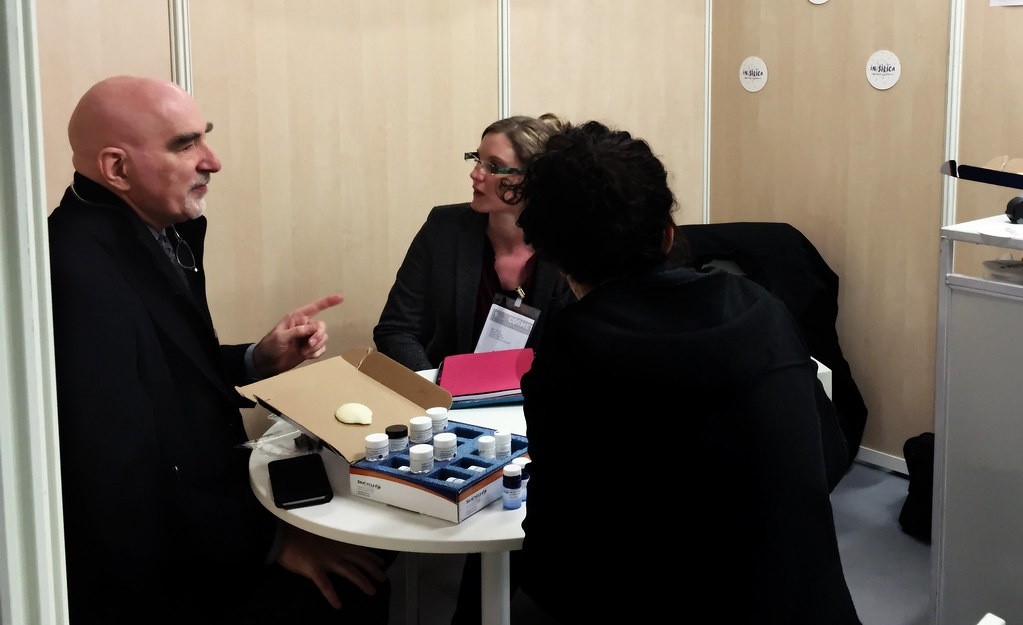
[982,259,1023,279]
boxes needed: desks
[247,368,526,625]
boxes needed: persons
[494,117,864,625]
[46,73,344,625]
[370,111,574,372]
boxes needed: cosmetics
[364,406,533,509]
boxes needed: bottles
[502,457,531,509]
[478,430,511,462]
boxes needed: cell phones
[268,453,334,510]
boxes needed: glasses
[171,224,198,272]
[464,152,525,177]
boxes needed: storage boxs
[235,346,532,523]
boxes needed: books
[438,347,535,403]
